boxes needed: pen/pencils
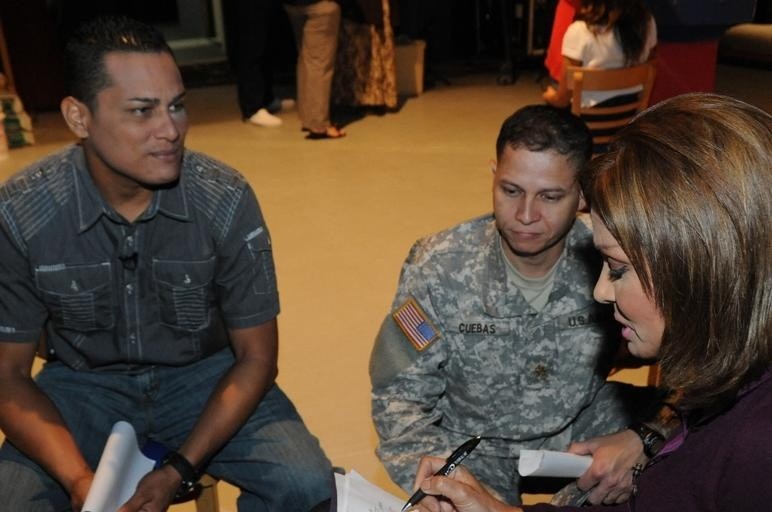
[401,435,483,512]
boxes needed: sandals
[306,127,345,140]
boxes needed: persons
[224,2,349,139]
[0,20,337,511]
[545,2,662,135]
[413,93,772,512]
[370,105,691,506]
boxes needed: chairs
[564,57,659,145]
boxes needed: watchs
[628,422,667,457]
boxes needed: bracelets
[167,452,202,487]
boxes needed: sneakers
[250,109,282,128]
[281,98,295,112]
